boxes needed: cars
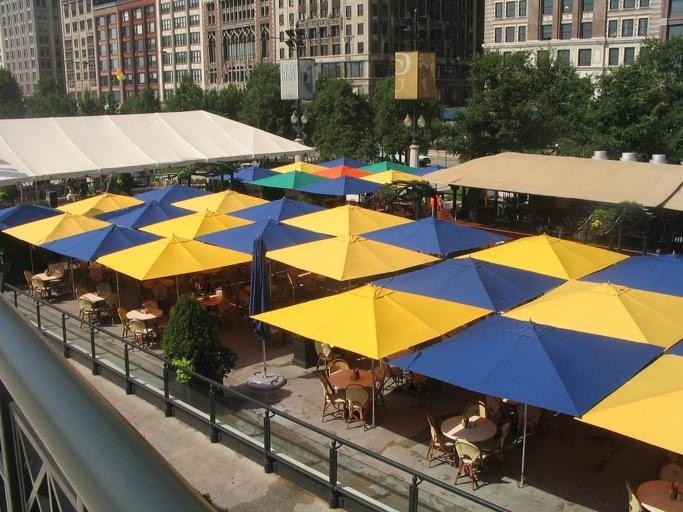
[387,149,431,167]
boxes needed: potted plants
[162,292,238,408]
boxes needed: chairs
[454,440,483,490]
[659,462,681,480]
[140,301,158,311]
[346,385,371,433]
[413,371,430,402]
[54,264,65,297]
[314,342,342,373]
[326,358,350,372]
[427,415,458,469]
[117,307,132,338]
[76,285,89,317]
[31,276,49,306]
[24,269,38,297]
[517,403,543,436]
[153,284,168,301]
[487,394,502,418]
[372,363,386,407]
[462,401,489,419]
[318,372,347,422]
[128,319,148,353]
[78,299,102,329]
[494,422,511,464]
[381,358,404,392]
[97,283,111,316]
[626,480,644,512]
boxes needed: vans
[439,105,467,121]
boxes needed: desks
[143,278,175,290]
[33,272,63,298]
[441,415,498,468]
[329,368,373,388]
[198,296,222,323]
[126,307,163,344]
[636,480,681,512]
[83,290,109,324]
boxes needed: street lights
[132,50,167,97]
[394,7,436,169]
[54,61,88,99]
[278,21,315,145]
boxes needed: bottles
[350,367,359,380]
[461,416,474,429]
[667,480,681,501]
